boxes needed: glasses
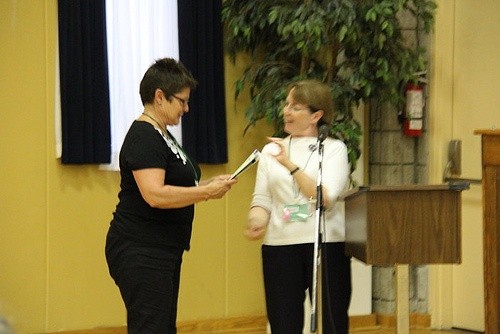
[173,94,188,107]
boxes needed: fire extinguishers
[397,71,428,138]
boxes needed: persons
[103,59,239,334]
[242,80,350,334]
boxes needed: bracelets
[291,167,299,175]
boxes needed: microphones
[319,126,329,142]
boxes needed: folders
[229,149,261,180]
